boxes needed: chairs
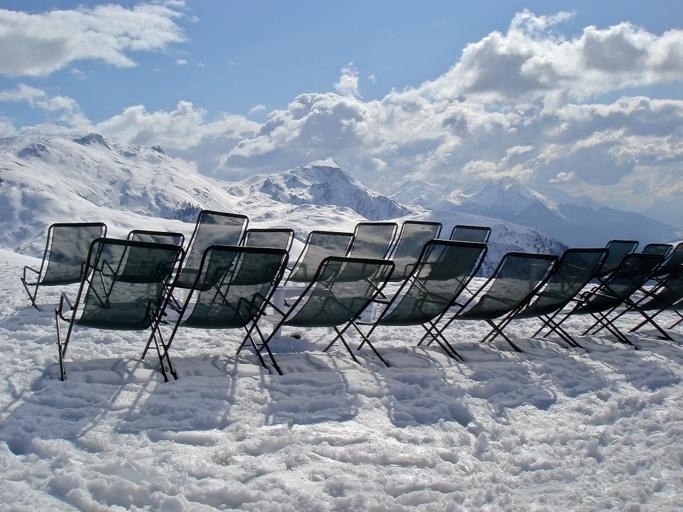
[161,211,248,316]
[402,223,492,311]
[355,218,444,303]
[544,237,682,348]
[211,225,296,332]
[346,238,489,370]
[152,243,290,380]
[19,220,107,313]
[245,254,397,377]
[54,236,187,382]
[97,228,185,327]
[480,247,609,355]
[273,229,364,322]
[411,250,561,365]
[319,221,400,319]
